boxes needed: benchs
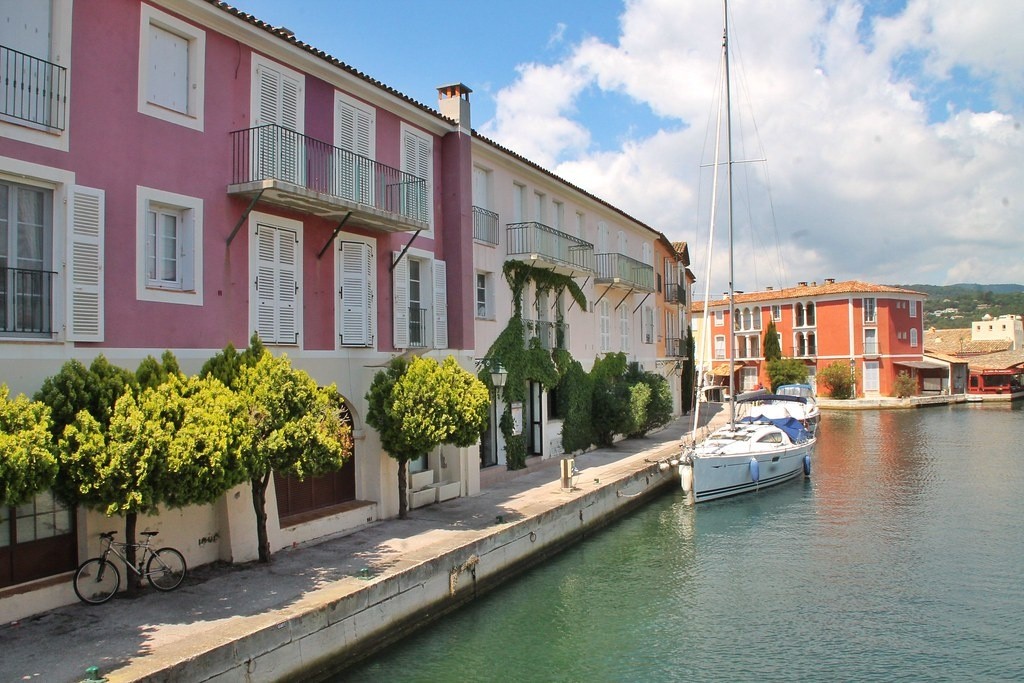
[722,389,740,402]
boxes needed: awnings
[706,363,744,376]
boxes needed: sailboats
[678,0,818,503]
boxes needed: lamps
[476,357,510,388]
[656,359,682,379]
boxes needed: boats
[771,383,821,432]
[965,367,1024,402]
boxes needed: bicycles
[73,531,187,605]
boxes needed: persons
[759,382,763,389]
